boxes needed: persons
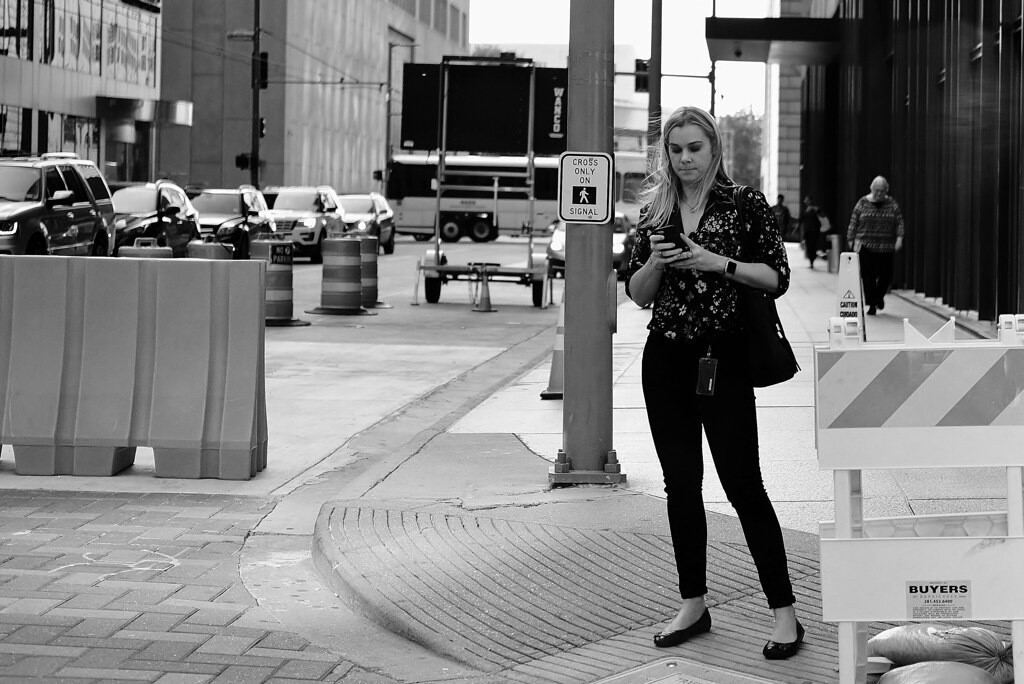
[792,197,823,268]
[625,106,805,659]
[847,175,904,315]
[771,195,790,240]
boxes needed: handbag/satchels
[736,327,801,387]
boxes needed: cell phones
[653,224,688,258]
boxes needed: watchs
[722,258,737,280]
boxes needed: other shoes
[867,307,876,315]
[878,298,885,311]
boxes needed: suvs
[263,186,345,264]
[340,192,396,255]
[183,184,275,258]
[0,151,116,254]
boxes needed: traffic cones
[538,291,564,398]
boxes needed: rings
[660,251,663,258]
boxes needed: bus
[387,152,647,243]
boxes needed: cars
[105,177,203,258]
[546,211,636,279]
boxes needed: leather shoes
[653,607,711,648]
[763,620,805,657]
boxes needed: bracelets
[650,255,665,271]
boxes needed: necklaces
[683,200,698,213]
